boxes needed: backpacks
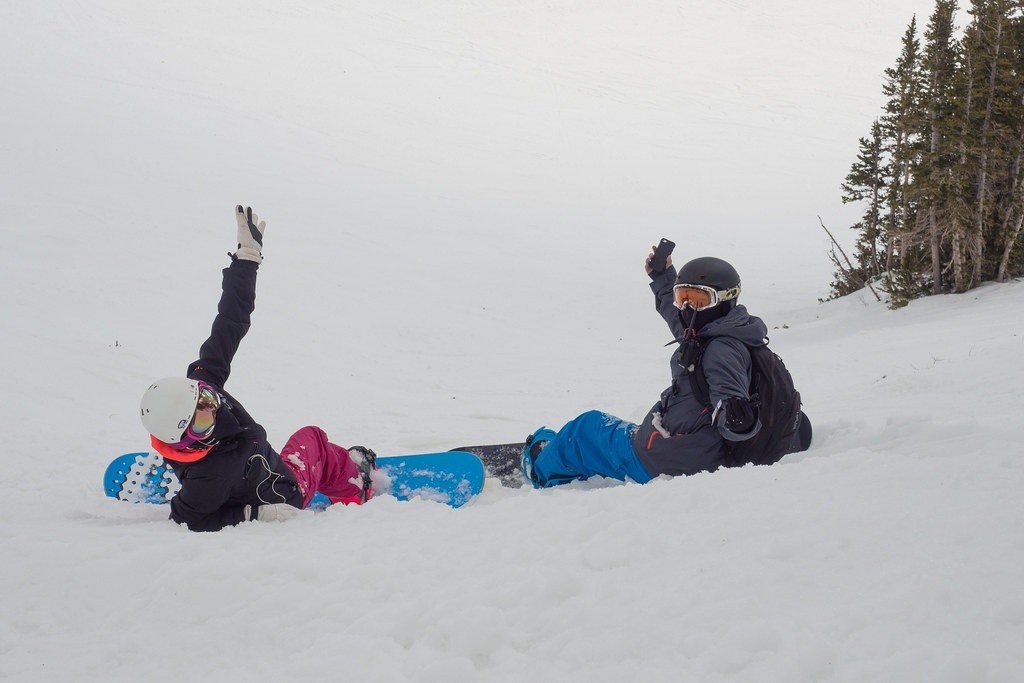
[687,333,804,471]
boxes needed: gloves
[229,204,267,265]
[245,502,317,524]
[722,395,756,433]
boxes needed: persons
[519,246,802,489]
[140,205,378,533]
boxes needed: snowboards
[449,408,814,491]
[103,450,488,513]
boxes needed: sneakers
[345,445,376,503]
[522,425,558,488]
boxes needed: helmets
[674,256,742,318]
[140,376,202,451]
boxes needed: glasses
[671,278,744,315]
[171,379,221,450]
